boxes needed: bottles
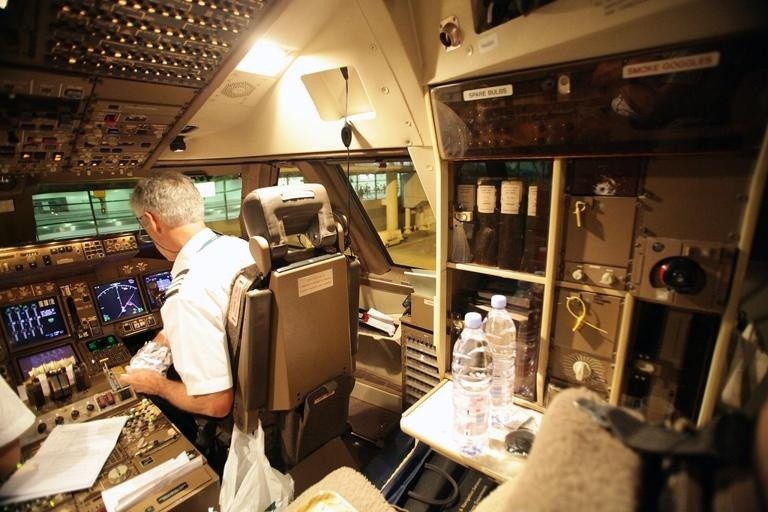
[481,294,517,428]
[453,312,492,449]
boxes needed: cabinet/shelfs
[433,158,759,431]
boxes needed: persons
[0,374,36,480]
[119,169,257,443]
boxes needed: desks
[400,382,546,488]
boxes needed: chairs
[194,182,362,474]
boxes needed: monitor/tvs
[0,296,71,354]
[143,272,173,311]
[16,345,80,377]
[90,275,148,325]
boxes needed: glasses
[136,211,152,229]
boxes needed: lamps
[169,135,186,153]
